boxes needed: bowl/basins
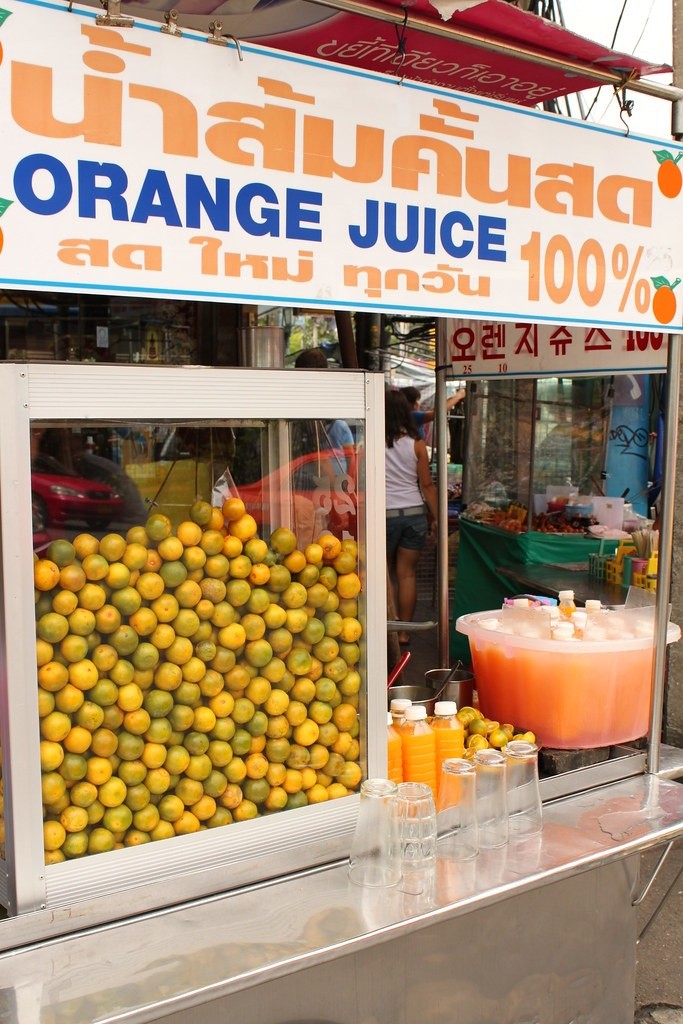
[455,608,681,750]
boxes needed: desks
[497,562,628,608]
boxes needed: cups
[438,757,479,862]
[347,779,405,889]
[387,684,442,714]
[426,667,476,707]
[398,781,438,873]
[502,741,542,836]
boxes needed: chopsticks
[631,528,659,560]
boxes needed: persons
[294,348,358,494]
[399,386,466,447]
[384,390,440,646]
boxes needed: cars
[230,444,357,543]
[30,450,123,532]
[429,419,611,501]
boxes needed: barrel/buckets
[564,504,594,517]
[547,502,565,510]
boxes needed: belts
[386,506,424,518]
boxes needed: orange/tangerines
[454,706,536,762]
[0,498,362,865]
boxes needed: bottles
[479,590,634,642]
[386,699,464,812]
[473,749,509,848]
